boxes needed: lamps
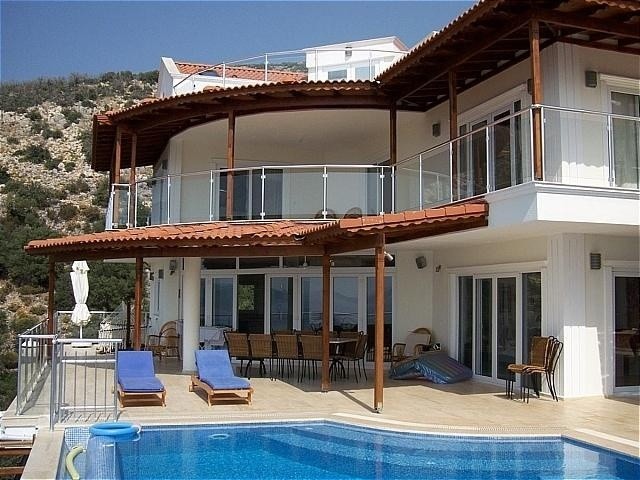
[384,251,394,262]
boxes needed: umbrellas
[70,260,94,339]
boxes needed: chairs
[145,320,181,362]
[223,326,369,384]
[505,332,564,404]
[111,349,254,408]
[389,327,474,385]
